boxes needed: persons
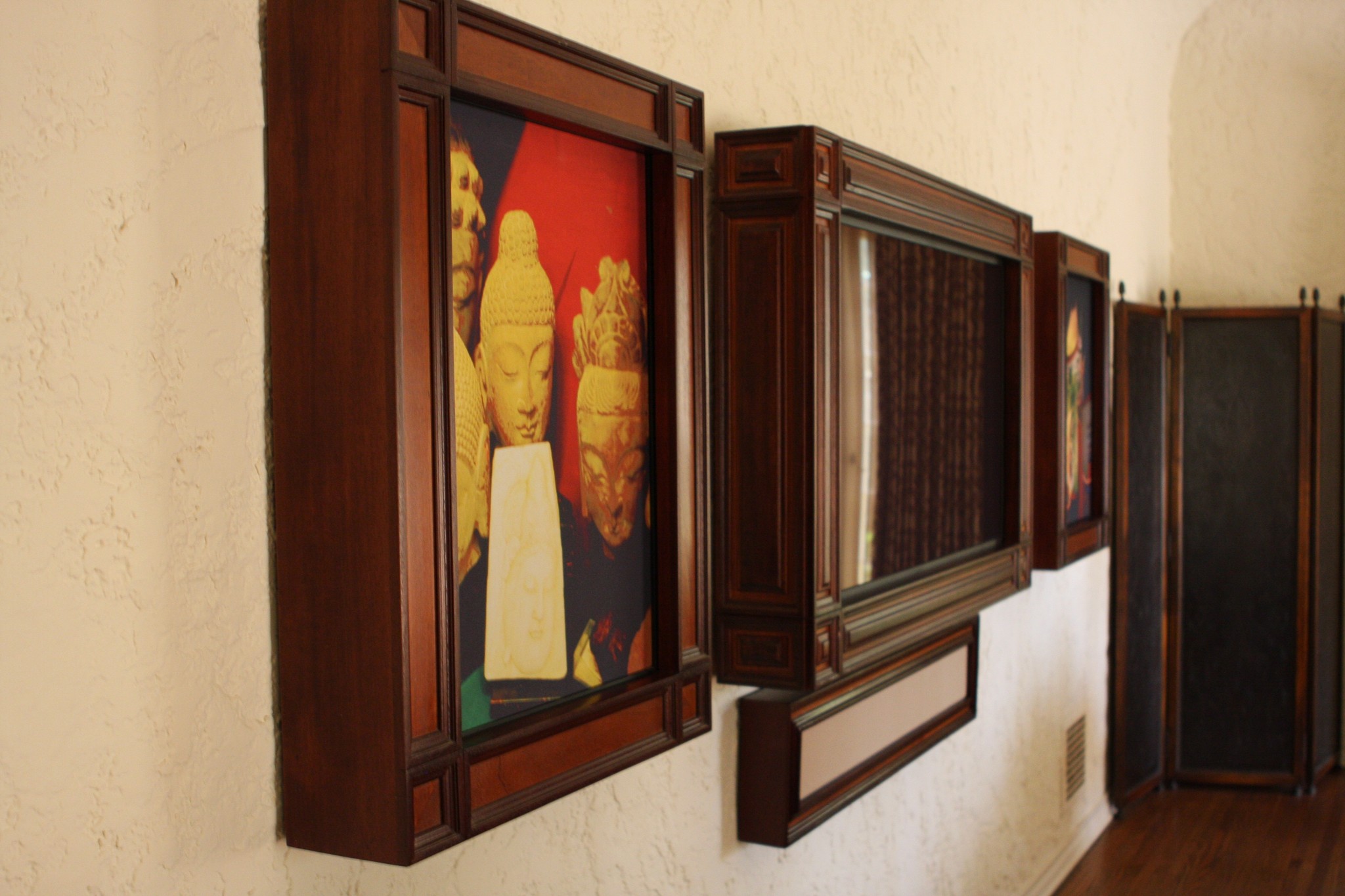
[475,211,557,446]
[448,146,653,731]
